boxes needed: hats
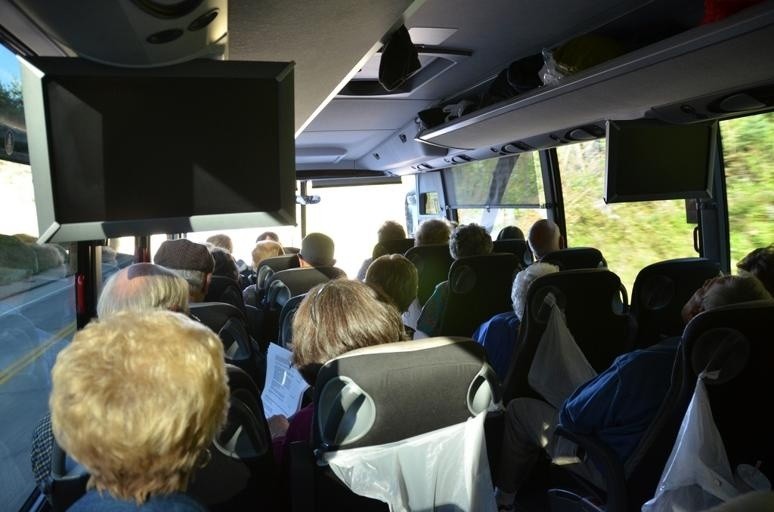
[154,239,215,273]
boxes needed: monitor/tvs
[19,52,299,246]
[602,117,719,205]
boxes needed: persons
[471,261,560,386]
[206,234,232,257]
[296,233,338,269]
[265,276,410,468]
[356,219,406,283]
[47,305,232,512]
[241,242,285,307]
[258,232,280,243]
[364,254,420,340]
[31,262,192,502]
[413,218,450,249]
[736,244,774,297]
[527,218,565,263]
[416,223,494,336]
[489,271,774,511]
[495,225,525,242]
[208,246,241,281]
[152,239,215,304]
